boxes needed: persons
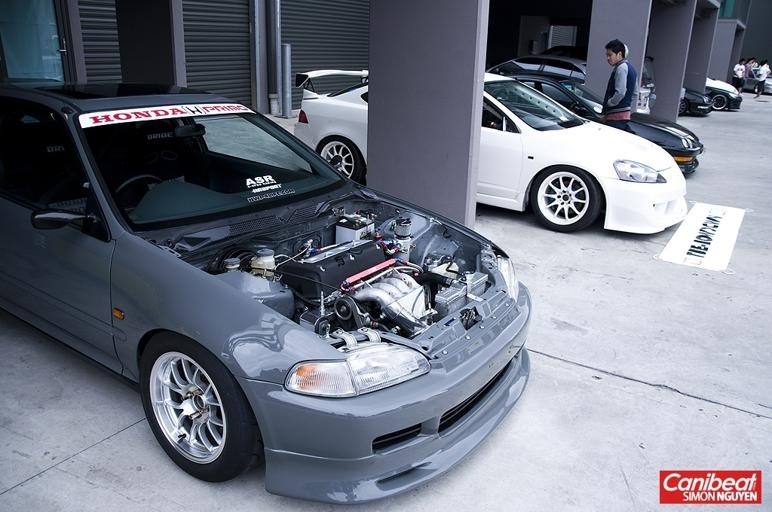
[732,56,772,99]
[601,39,633,131]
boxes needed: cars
[1,75,536,508]
[289,45,742,239]
[743,68,772,94]
